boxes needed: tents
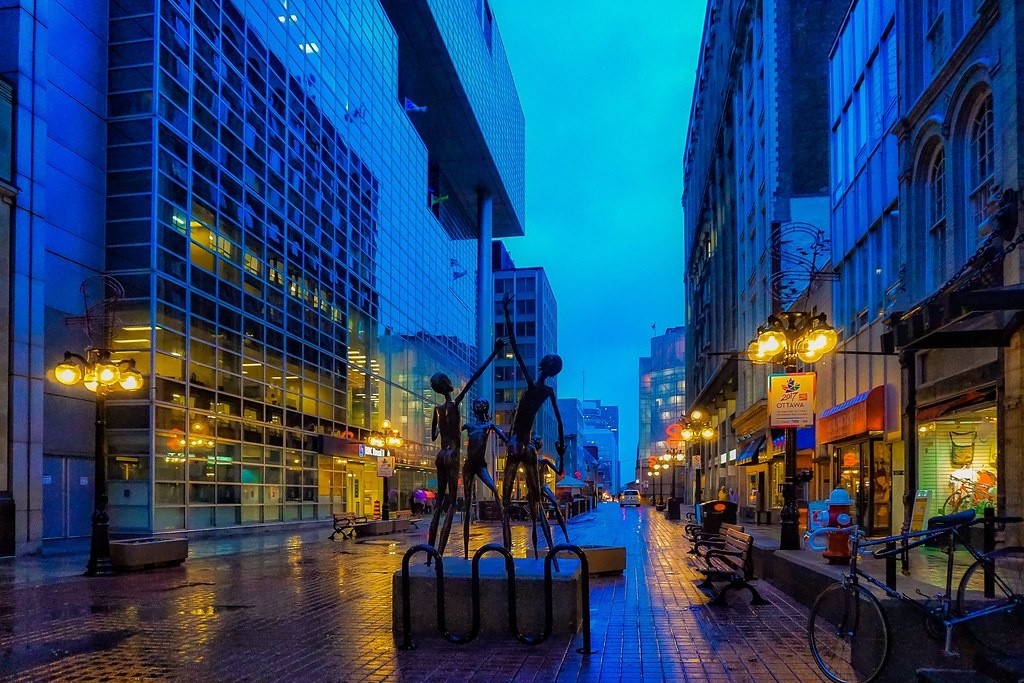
[556,475,588,499]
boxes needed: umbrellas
[414,490,426,501]
[424,491,435,500]
[388,490,398,497]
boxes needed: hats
[720,486,727,490]
[729,488,735,492]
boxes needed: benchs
[689,527,771,607]
[396,509,423,529]
[694,522,745,557]
[327,512,371,542]
[682,523,711,554]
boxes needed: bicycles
[805,507,1024,682]
[943,478,998,515]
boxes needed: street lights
[368,420,404,522]
[682,411,715,518]
[648,468,660,507]
[662,443,683,500]
[654,455,670,505]
[746,310,838,558]
[56,344,147,575]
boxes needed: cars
[619,490,642,507]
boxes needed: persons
[718,486,738,503]
[425,290,569,573]
[409,488,463,515]
[389,488,413,511]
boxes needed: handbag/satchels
[950,431,977,465]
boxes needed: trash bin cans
[696,499,738,549]
[666,497,681,521]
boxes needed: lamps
[741,431,747,442]
[735,434,741,444]
[747,427,756,438]
